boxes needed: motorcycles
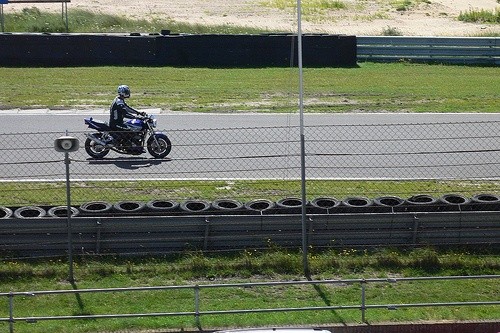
[83,111,172,160]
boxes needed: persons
[108,84,143,149]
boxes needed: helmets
[118,85,131,98]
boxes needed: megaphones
[53,135,79,153]
